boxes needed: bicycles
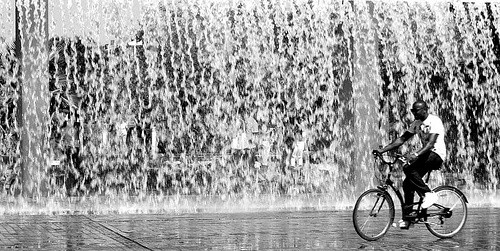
[352,151,468,241]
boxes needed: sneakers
[421,191,439,209]
[398,213,413,227]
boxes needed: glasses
[410,107,426,113]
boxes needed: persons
[373,100,446,230]
